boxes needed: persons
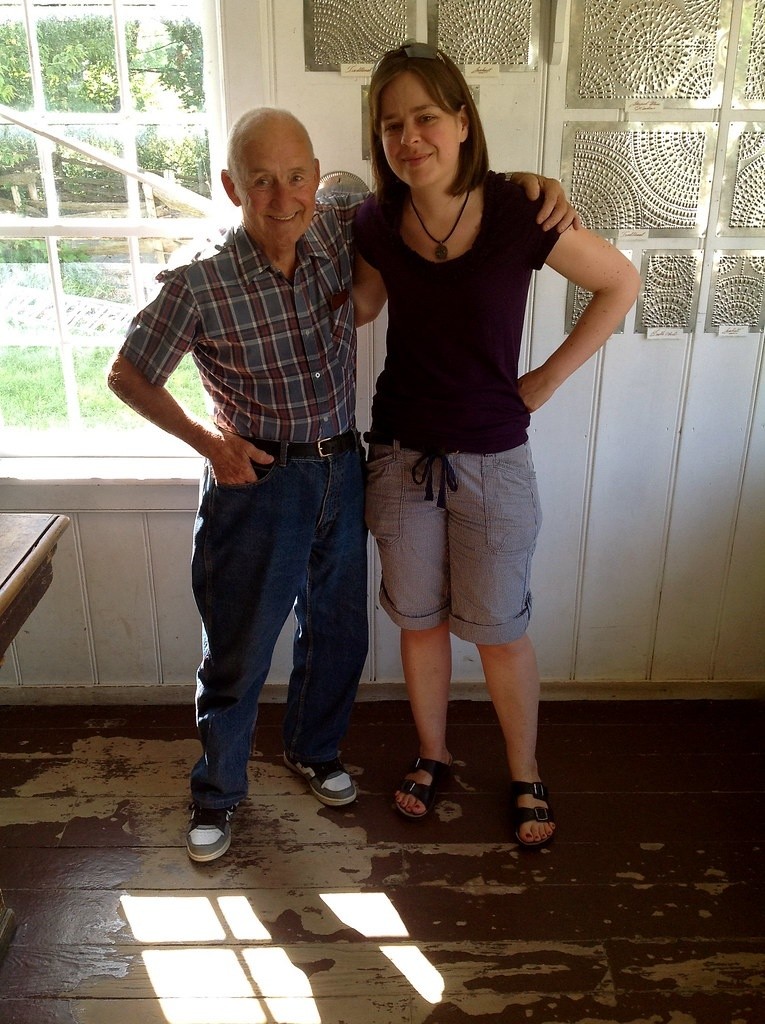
[107,108,581,862]
[349,45,642,850]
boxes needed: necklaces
[410,192,470,261]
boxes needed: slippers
[394,752,453,822]
[510,780,557,850]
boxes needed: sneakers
[284,751,356,807]
[186,801,239,862]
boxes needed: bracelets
[505,172,515,182]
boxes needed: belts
[241,427,361,458]
[363,431,428,453]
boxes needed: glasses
[371,43,445,79]
